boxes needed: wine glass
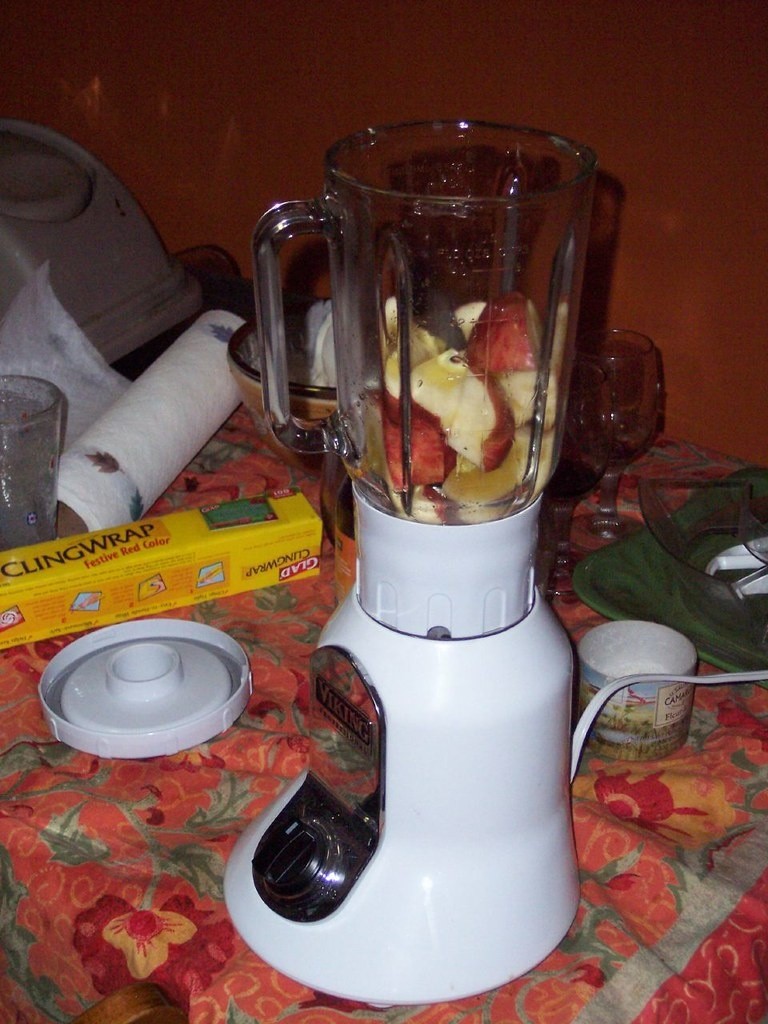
[572,327,657,554]
[532,359,603,599]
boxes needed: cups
[0,376,69,554]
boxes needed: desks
[0,318,768,1024]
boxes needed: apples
[372,287,573,528]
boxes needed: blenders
[223,112,599,1009]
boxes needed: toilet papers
[0,258,250,536]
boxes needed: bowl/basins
[226,307,340,477]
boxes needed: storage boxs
[0,483,323,651]
